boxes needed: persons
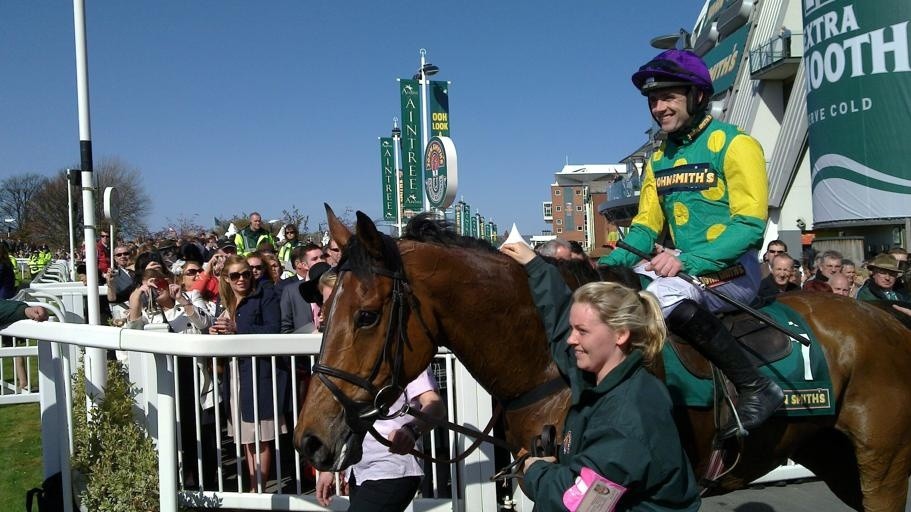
[596,49,786,450]
[778,25,792,58]
[1,214,446,511]
[614,173,623,182]
[496,238,910,511]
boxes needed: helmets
[631,47,714,116]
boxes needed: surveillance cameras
[796,219,806,232]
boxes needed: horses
[292,201,911,512]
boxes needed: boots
[673,305,788,448]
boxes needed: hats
[299,262,331,303]
[866,253,905,274]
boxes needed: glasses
[767,249,783,253]
[100,230,339,281]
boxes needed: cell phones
[897,260,910,270]
